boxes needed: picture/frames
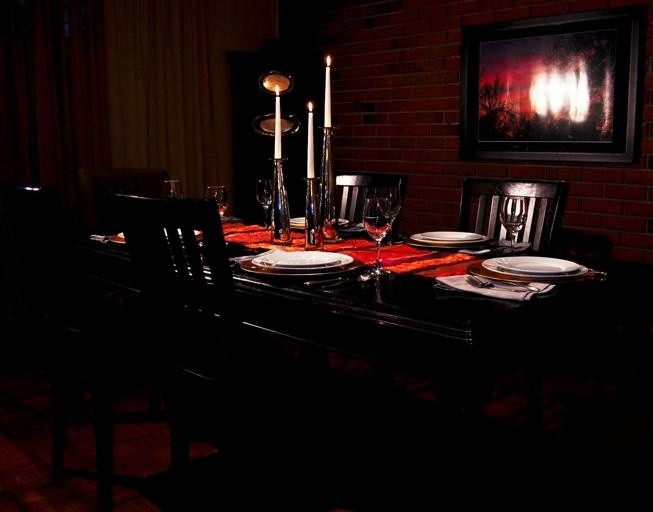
[460,5,647,163]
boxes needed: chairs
[455,177,570,251]
[114,193,301,507]
[1,184,239,508]
[335,174,409,232]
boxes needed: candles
[273,83,282,158]
[307,100,315,177]
[323,54,333,128]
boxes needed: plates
[251,249,355,270]
[481,256,589,278]
[290,216,349,228]
[409,230,489,245]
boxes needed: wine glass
[363,197,404,276]
[499,195,526,255]
[203,186,231,220]
[375,187,402,245]
[255,178,276,229]
[161,180,184,200]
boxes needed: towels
[433,274,556,301]
